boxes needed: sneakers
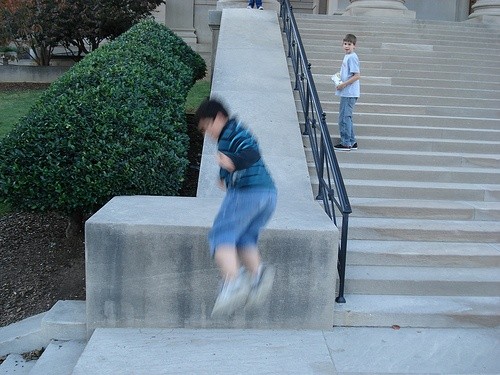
[334,143,359,151]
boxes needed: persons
[247,0,263,10]
[193,101,278,318]
[334,34,362,151]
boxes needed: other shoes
[250,265,275,306]
[246,6,253,9]
[212,278,250,315]
[258,6,264,9]
